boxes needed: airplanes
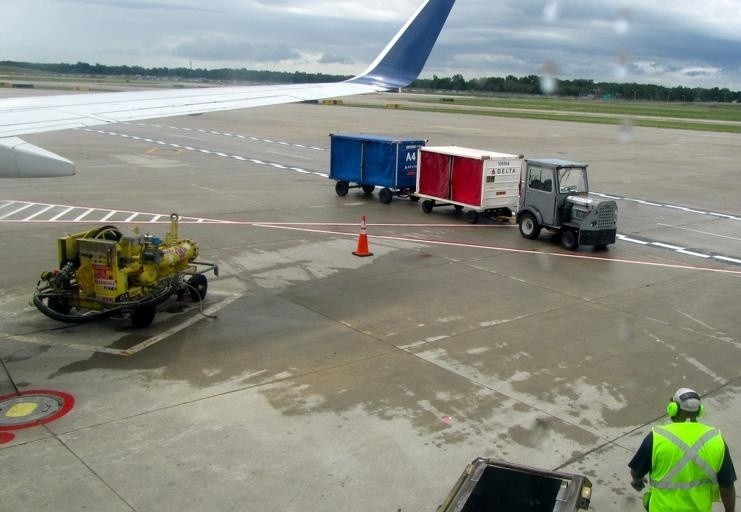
[0,0,457,178]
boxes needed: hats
[672,387,700,412]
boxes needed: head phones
[666,392,705,417]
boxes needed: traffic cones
[352,216,374,257]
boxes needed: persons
[625,387,739,512]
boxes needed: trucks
[329,132,617,250]
